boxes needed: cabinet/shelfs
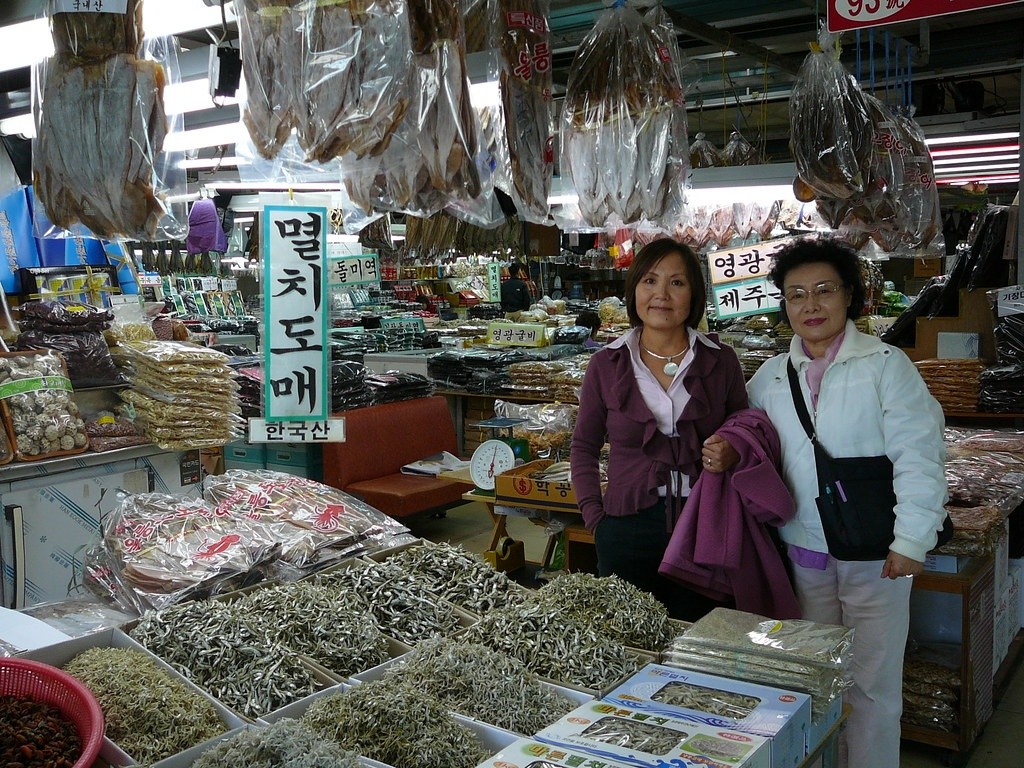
[901,553,997,753]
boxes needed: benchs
[317,395,477,522]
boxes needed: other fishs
[199,467,417,584]
[81,488,283,618]
[560,6,689,229]
[237,0,412,164]
[338,1,553,228]
[61,538,759,768]
[30,0,172,242]
[788,54,941,253]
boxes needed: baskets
[0,658,104,768]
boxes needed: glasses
[782,282,845,304]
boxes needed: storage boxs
[201,433,321,487]
[494,459,609,513]
[19,539,842,768]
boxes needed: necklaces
[639,335,689,377]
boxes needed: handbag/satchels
[814,455,954,560]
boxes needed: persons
[745,237,951,768]
[568,236,751,622]
[500,264,532,312]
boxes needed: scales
[469,417,534,497]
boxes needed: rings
[905,573,913,578]
[708,457,712,465]
